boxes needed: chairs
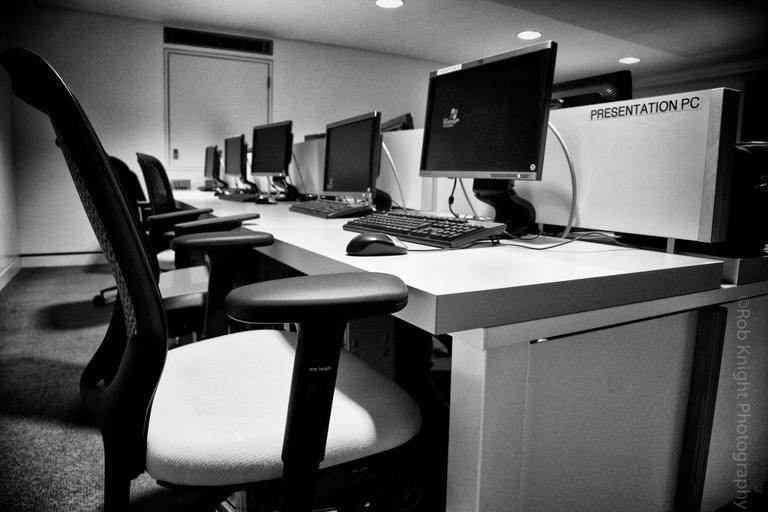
[2,42,766,512]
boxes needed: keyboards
[342,209,507,249]
[289,198,372,218]
[219,188,267,202]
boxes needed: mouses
[255,195,277,204]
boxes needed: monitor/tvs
[547,69,633,110]
[199,145,228,192]
[251,120,298,202]
[223,133,259,194]
[303,134,326,142]
[418,40,558,244]
[321,111,391,219]
[381,113,414,132]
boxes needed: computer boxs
[698,139,768,259]
